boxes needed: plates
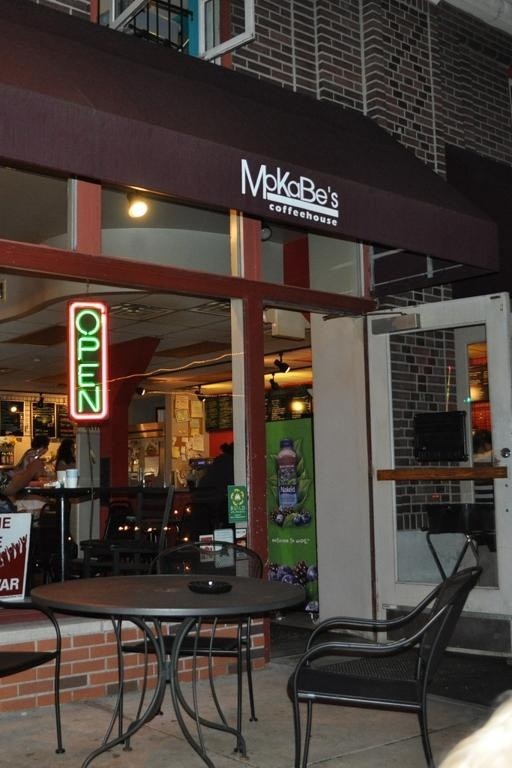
[188,581,232,595]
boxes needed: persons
[13,435,51,481]
[201,442,235,532]
[56,439,76,477]
[0,456,41,593]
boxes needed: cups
[57,467,80,490]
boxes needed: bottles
[276,437,300,508]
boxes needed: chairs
[121,541,264,754]
[32,500,76,573]
[287,565,484,768]
[78,487,175,578]
[0,599,65,754]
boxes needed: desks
[27,485,203,582]
[420,502,495,585]
[31,576,307,768]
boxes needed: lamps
[137,387,146,396]
[261,229,273,240]
[10,389,46,414]
[128,196,149,219]
[267,354,290,397]
[195,386,207,402]
